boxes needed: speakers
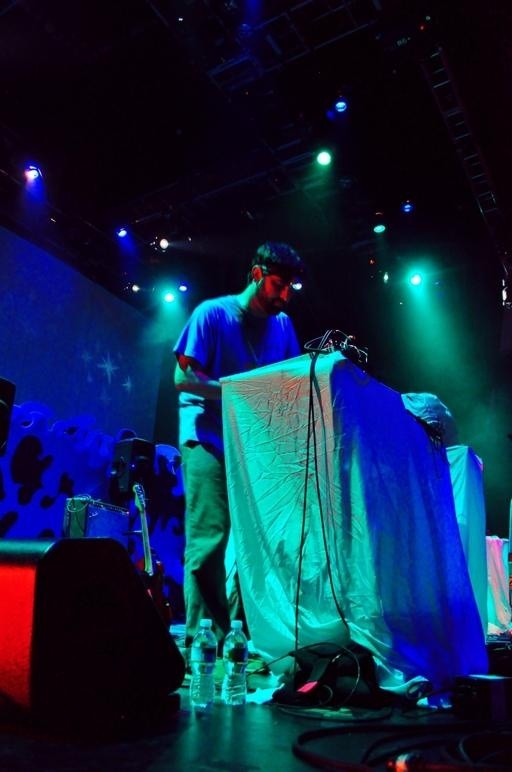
[0,537,185,718]
[109,438,155,498]
[0,378,16,455]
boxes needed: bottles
[190,611,250,710]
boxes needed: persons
[171,239,304,691]
